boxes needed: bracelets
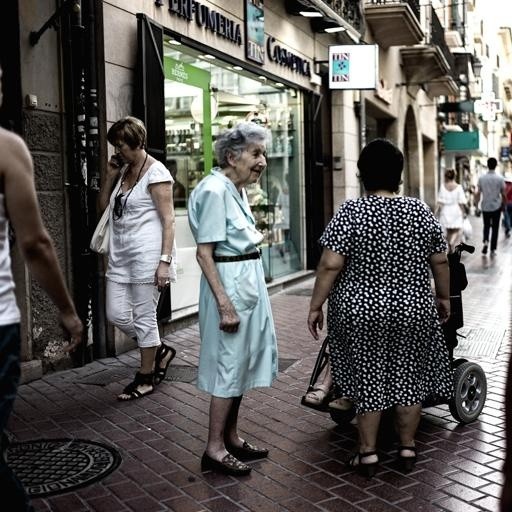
[159,254,173,265]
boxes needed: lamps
[282,0,327,20]
[305,15,348,38]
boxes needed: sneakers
[481,244,497,257]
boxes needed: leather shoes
[328,395,358,409]
[304,383,330,405]
[200,449,253,477]
[395,445,418,472]
[344,451,379,477]
[225,442,270,461]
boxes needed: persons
[434,158,512,256]
[306,136,451,481]
[304,273,437,411]
[0,67,84,507]
[186,122,278,478]
[98,115,177,404]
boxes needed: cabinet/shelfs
[158,114,298,285]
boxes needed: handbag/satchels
[89,200,111,255]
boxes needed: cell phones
[110,155,125,168]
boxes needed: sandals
[116,371,155,402]
[151,341,177,385]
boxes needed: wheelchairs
[296,236,495,427]
